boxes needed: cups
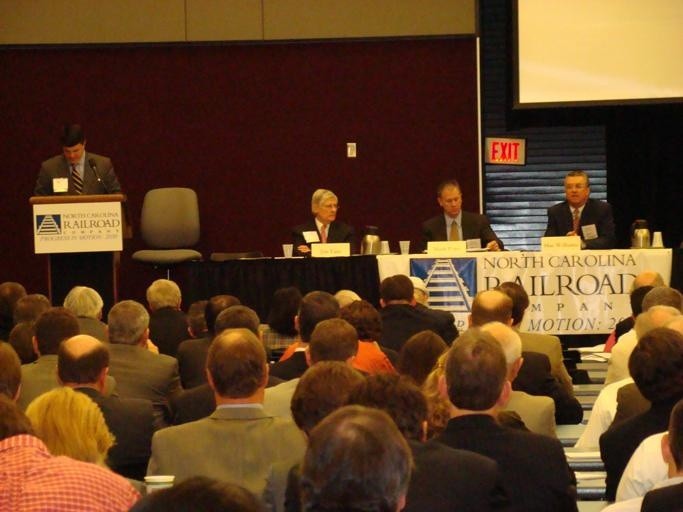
[380,241,390,255]
[282,244,293,257]
[399,240,411,254]
[144,475,175,494]
[652,231,664,248]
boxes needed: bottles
[360,226,381,255]
[629,219,651,248]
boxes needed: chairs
[211,251,263,263]
[130,186,203,268]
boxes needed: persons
[416,178,505,253]
[33,121,122,196]
[293,188,357,256]
[1,269,683,511]
[544,169,617,248]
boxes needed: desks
[557,361,614,512]
[220,247,673,335]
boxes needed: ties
[320,225,327,243]
[449,220,460,241]
[69,161,83,196]
[573,208,579,234]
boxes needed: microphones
[88,157,103,183]
